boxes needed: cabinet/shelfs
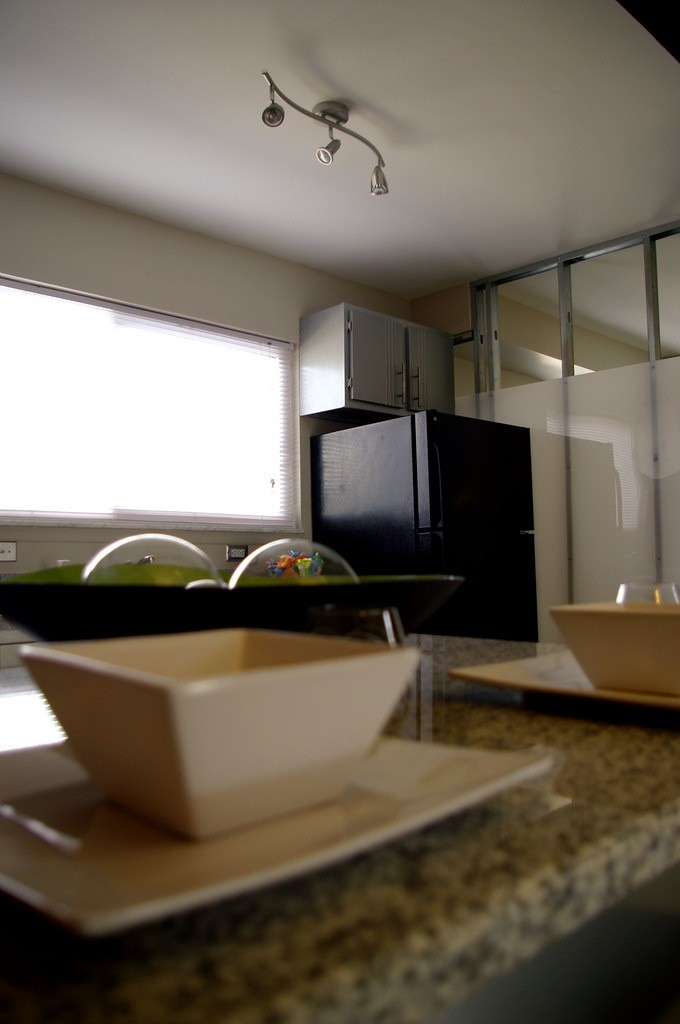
[311,410,538,642]
[299,304,453,420]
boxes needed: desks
[0,632,680,1024]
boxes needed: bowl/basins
[548,602,680,697]
[21,630,420,838]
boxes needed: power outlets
[226,545,247,561]
[0,540,17,562]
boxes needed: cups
[615,583,679,603]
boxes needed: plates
[446,650,680,712]
[0,729,554,940]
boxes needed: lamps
[257,71,392,195]
